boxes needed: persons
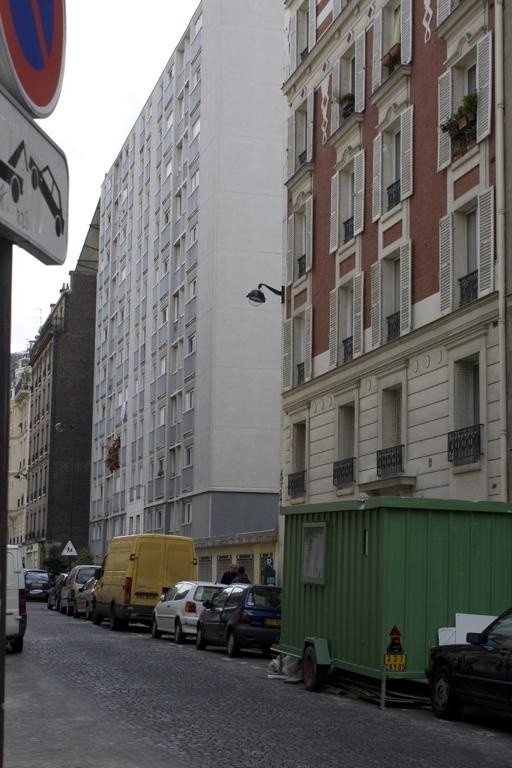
[231,567,252,584]
[47,582,56,609]
[221,564,239,584]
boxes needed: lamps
[14,471,28,480]
[247,284,285,303]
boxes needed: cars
[422,601,512,724]
[24,564,278,656]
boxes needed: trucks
[87,531,207,637]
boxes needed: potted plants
[339,94,354,109]
[441,94,476,161]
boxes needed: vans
[5,543,28,660]
